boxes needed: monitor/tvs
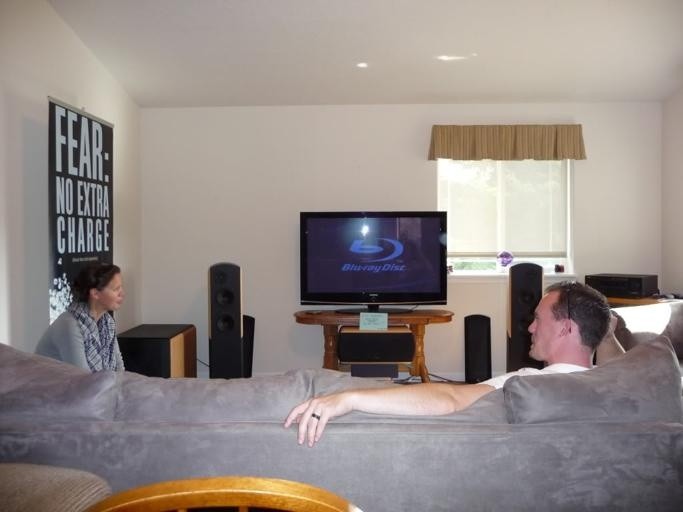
[299,210,447,312]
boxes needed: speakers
[463,314,492,385]
[207,263,242,379]
[505,263,544,371]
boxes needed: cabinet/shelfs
[116,324,196,377]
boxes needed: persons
[283,280,627,448]
[34,260,126,375]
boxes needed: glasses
[560,277,577,336]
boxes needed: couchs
[0,331,682,512]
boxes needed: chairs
[81,477,361,512]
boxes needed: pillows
[0,342,147,422]
[312,367,502,424]
[503,335,682,424]
[115,368,310,424]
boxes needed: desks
[604,293,670,310]
[294,308,454,382]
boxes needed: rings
[311,413,321,420]
